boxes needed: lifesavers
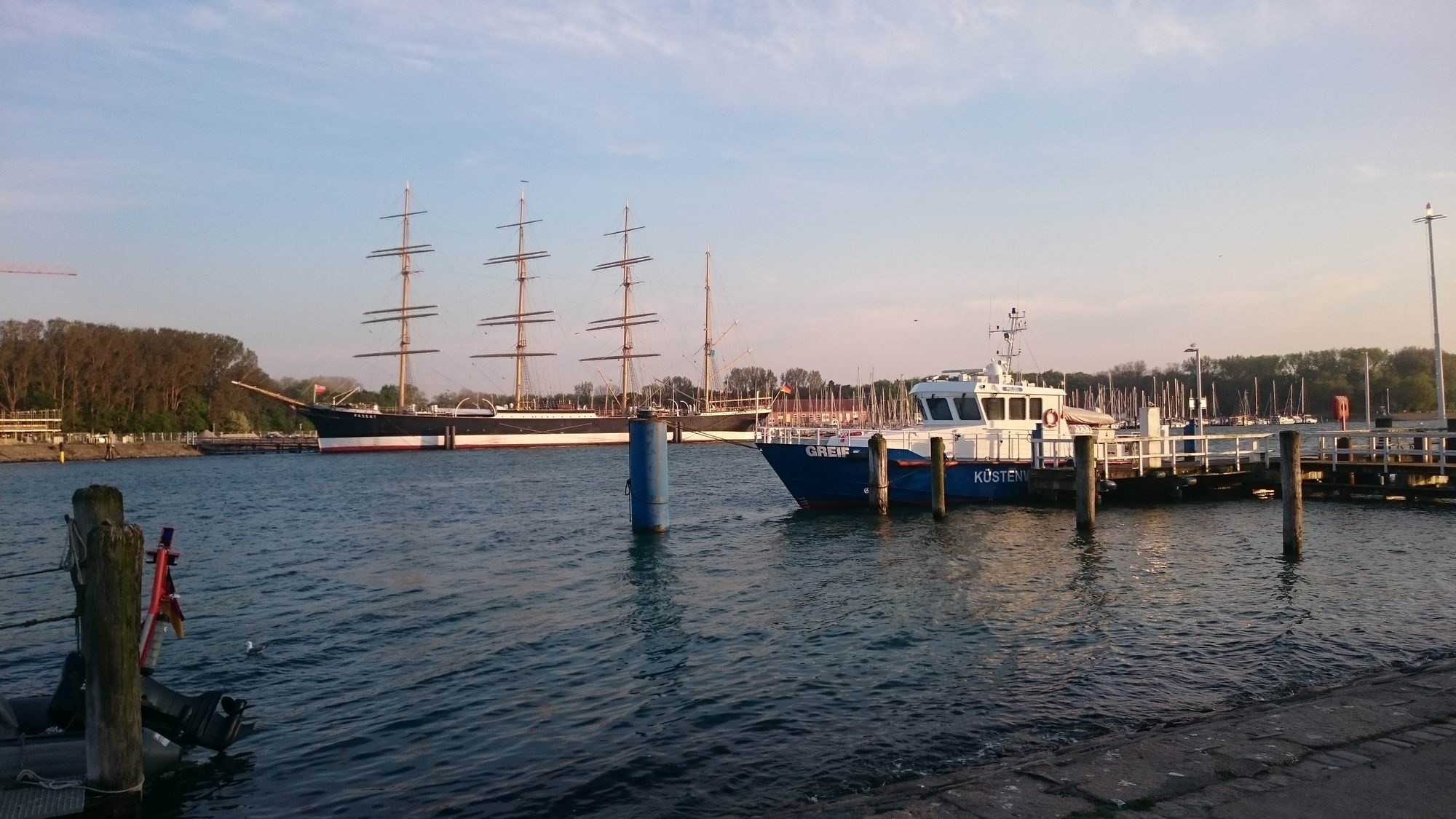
[1045,409,1059,427]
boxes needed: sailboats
[228,178,775,452]
[1106,370,1321,431]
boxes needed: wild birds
[244,641,269,654]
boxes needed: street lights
[1182,348,1205,466]
[1413,203,1447,429]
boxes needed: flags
[315,385,326,393]
[781,382,792,394]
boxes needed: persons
[629,405,632,412]
[509,402,513,409]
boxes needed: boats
[756,304,1171,520]
